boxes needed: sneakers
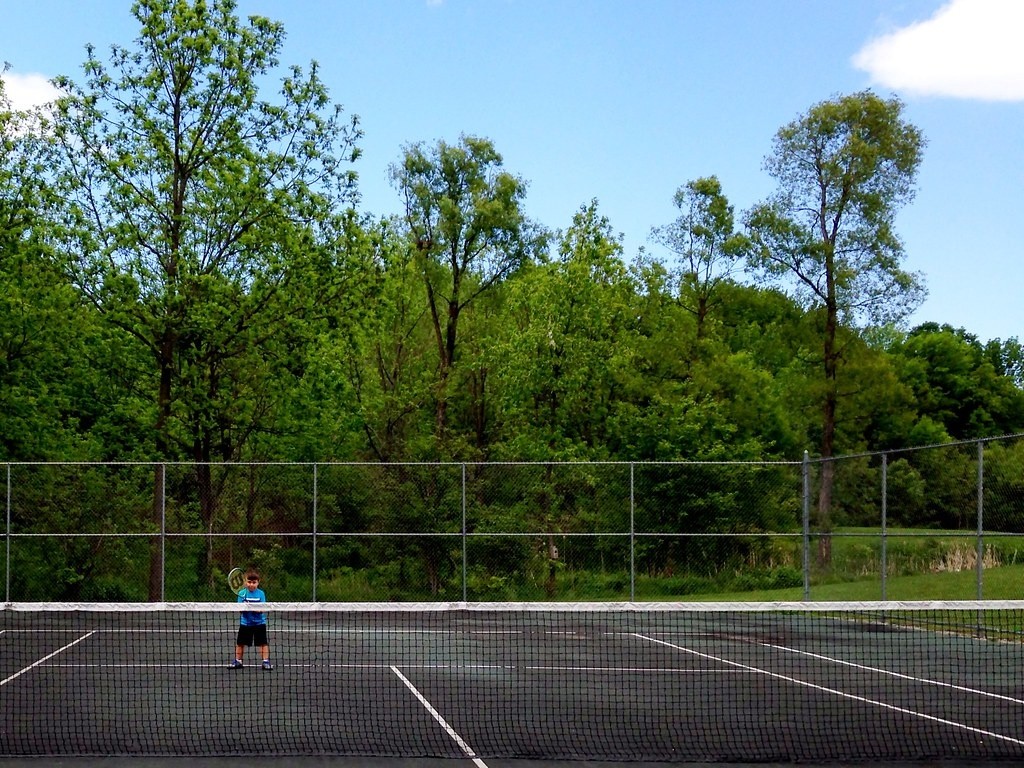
[262,661,274,670]
[226,659,244,669]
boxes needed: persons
[227,568,271,669]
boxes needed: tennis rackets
[227,567,248,600]
